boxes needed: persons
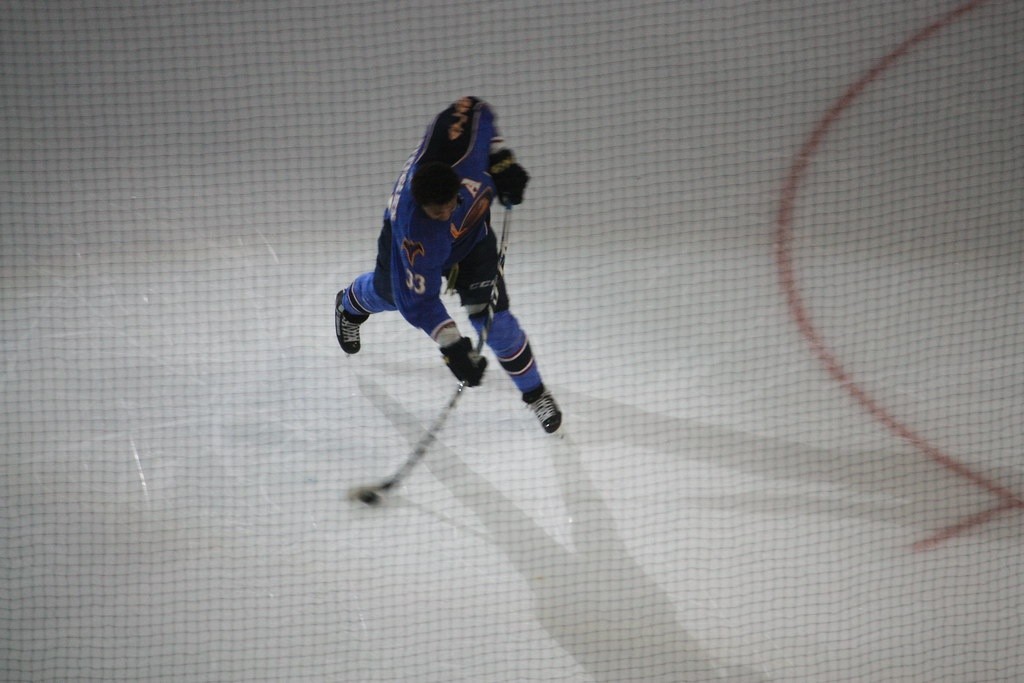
[334,96,563,440]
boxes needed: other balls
[357,489,381,507]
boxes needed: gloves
[440,336,487,388]
[486,147,531,206]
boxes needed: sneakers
[529,390,563,436]
[334,288,366,358]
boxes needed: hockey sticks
[345,202,514,510]
